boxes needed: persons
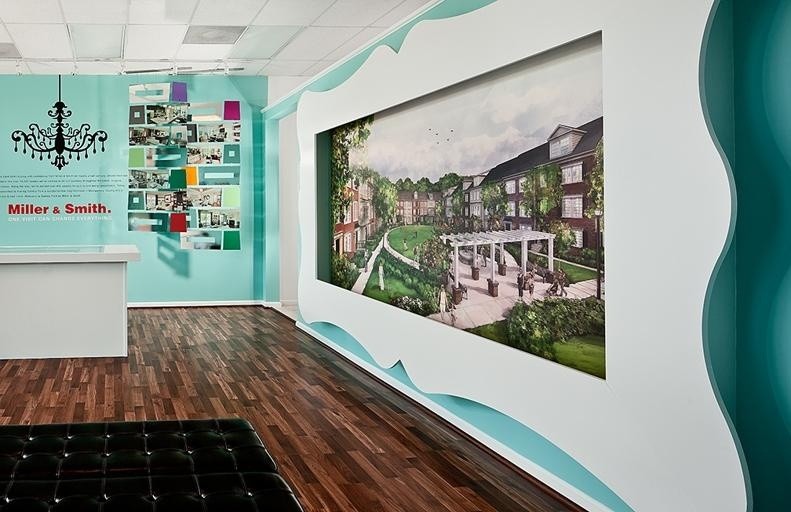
[375,215,567,326]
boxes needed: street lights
[591,206,604,300]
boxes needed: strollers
[544,280,560,295]
